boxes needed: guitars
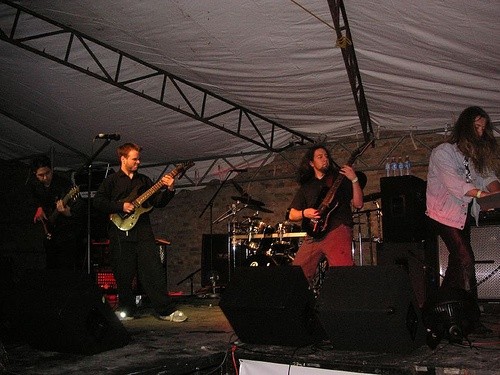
[108,160,196,233]
[35,185,79,240]
[301,133,376,239]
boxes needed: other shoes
[467,326,494,337]
[113,311,134,320]
[160,310,188,322]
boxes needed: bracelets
[302,210,305,218]
[477,191,482,198]
[352,177,358,183]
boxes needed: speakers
[27,282,131,354]
[201,234,234,289]
[218,175,500,351]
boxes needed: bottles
[384,155,409,177]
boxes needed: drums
[242,221,301,251]
[243,254,291,268]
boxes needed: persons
[287,145,364,286]
[92,142,189,323]
[27,155,84,266]
[424,106,500,336]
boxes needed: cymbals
[231,196,275,214]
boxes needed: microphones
[230,169,248,172]
[96,133,120,141]
